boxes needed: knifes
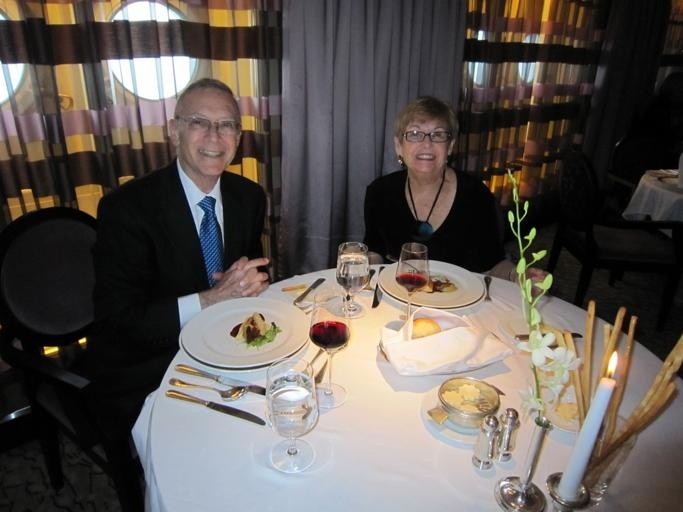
[293,278,326,306]
[342,269,376,303]
[175,364,266,396]
[165,389,266,426]
[516,330,583,342]
[371,265,387,310]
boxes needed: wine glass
[265,355,320,475]
[395,241,431,322]
[327,240,371,321]
[307,286,351,412]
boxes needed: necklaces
[406,169,446,242]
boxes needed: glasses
[179,115,242,136]
[402,129,453,144]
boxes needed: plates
[419,383,505,452]
[379,259,487,313]
[177,295,312,374]
[499,312,565,354]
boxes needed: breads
[412,318,439,339]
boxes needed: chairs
[545,141,683,311]
[1,208,129,512]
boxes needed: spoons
[169,377,246,403]
[484,274,493,304]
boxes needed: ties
[197,197,225,289]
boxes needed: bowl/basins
[425,378,506,429]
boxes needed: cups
[584,413,637,506]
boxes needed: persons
[363,96,509,273]
[96,78,268,374]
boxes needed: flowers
[505,170,581,425]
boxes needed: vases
[493,419,552,512]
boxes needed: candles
[559,350,616,501]
